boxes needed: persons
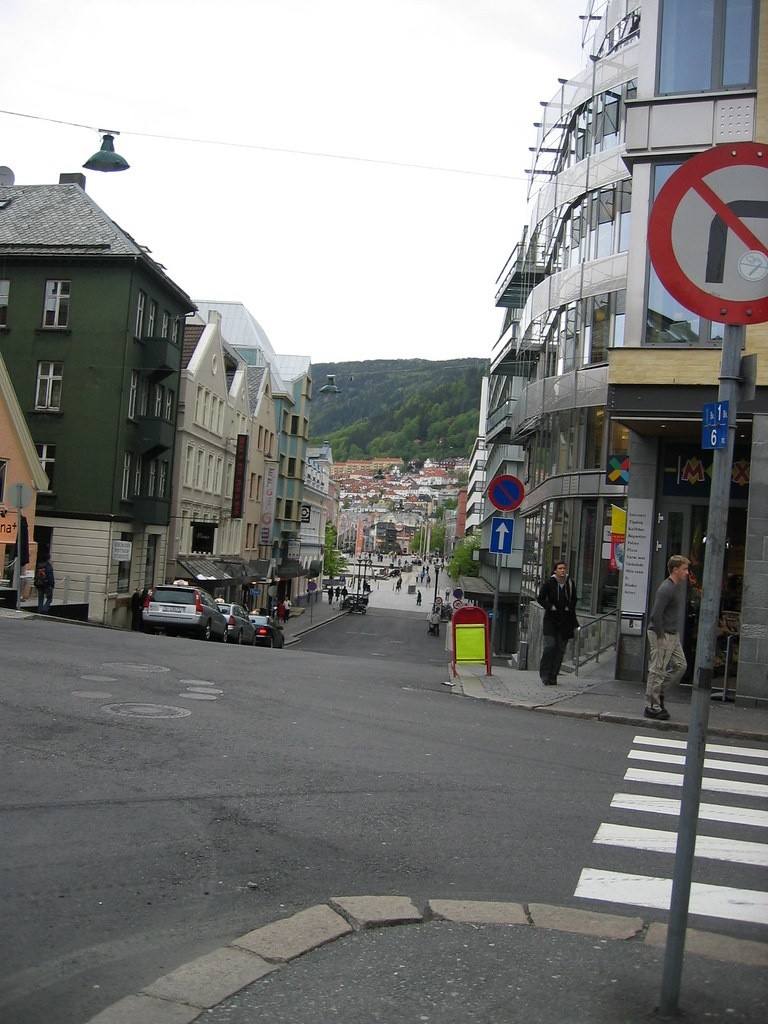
[537,560,581,686]
[277,596,292,623]
[35,554,55,615]
[328,550,473,636]
[644,554,688,720]
[131,587,155,633]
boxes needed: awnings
[173,556,262,588]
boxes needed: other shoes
[645,706,669,720]
[542,677,557,685]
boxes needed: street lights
[356,555,363,594]
[362,556,367,594]
[434,564,439,603]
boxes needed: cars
[249,615,284,649]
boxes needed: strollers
[427,622,435,635]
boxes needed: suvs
[142,586,228,643]
[216,603,256,646]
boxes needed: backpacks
[34,564,49,589]
